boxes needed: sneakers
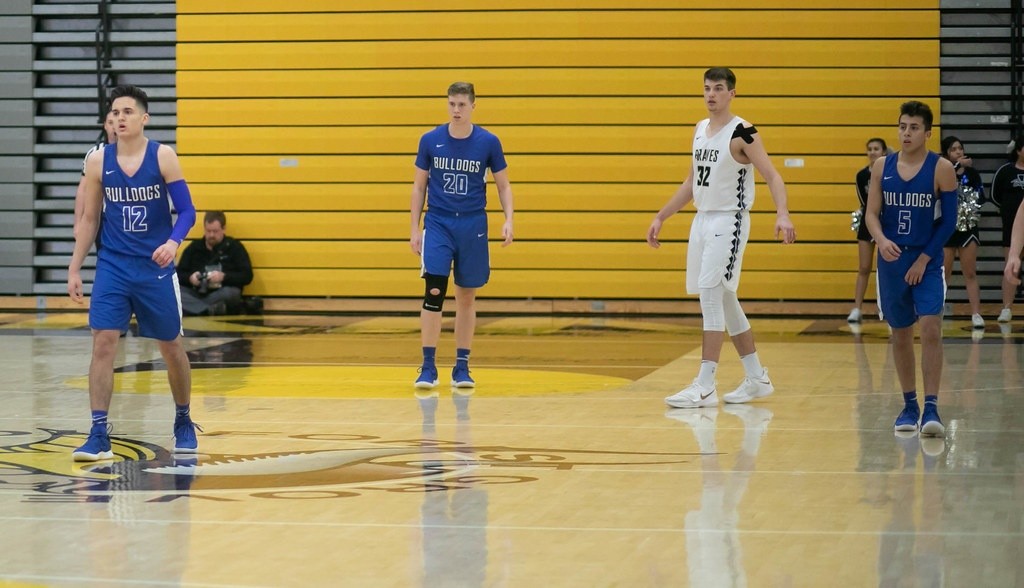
[664,377,719,408]
[998,323,1011,334]
[847,308,862,322]
[723,366,774,403]
[72,422,115,462]
[74,462,113,492]
[450,366,475,388]
[171,415,205,453]
[920,438,946,458]
[451,388,475,402]
[415,391,440,411]
[894,432,919,447]
[893,408,921,431]
[665,408,719,427]
[997,308,1012,322]
[723,404,774,433]
[972,313,985,328]
[971,329,984,341]
[920,411,945,434]
[414,361,440,389]
[172,455,198,483]
[851,323,861,333]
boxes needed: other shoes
[207,302,227,315]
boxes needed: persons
[411,81,513,389]
[646,66,797,409]
[847,135,1024,328]
[865,100,958,435]
[75,100,264,338]
[68,85,206,462]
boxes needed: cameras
[197,272,210,295]
